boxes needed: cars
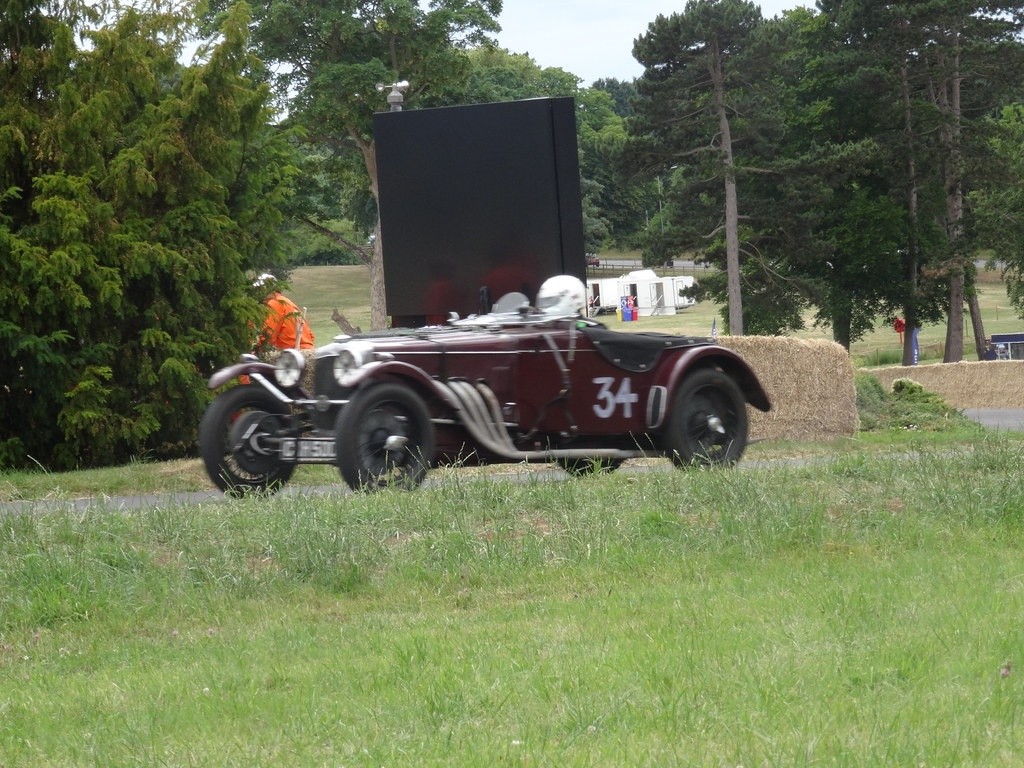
[197,309,773,502]
[586,252,599,267]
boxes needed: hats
[253,274,278,286]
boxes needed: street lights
[657,166,677,234]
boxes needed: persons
[536,274,587,316]
[228,273,314,421]
[589,296,594,308]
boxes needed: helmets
[536,275,586,314]
